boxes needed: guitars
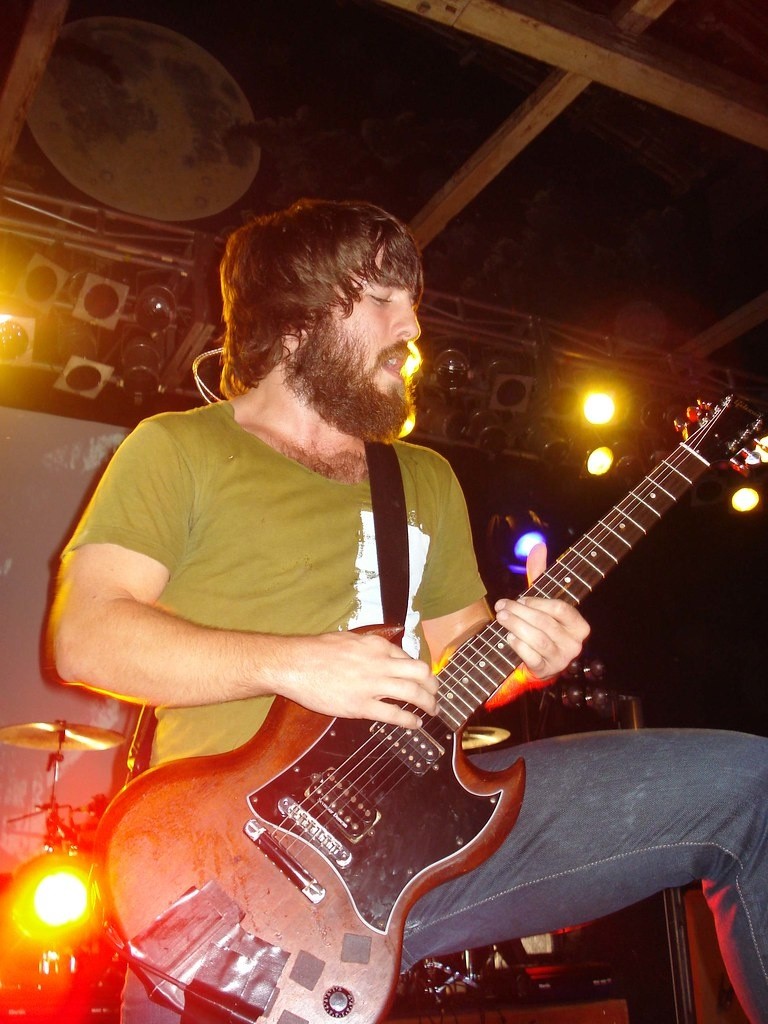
[96,393,768,1023]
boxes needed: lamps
[543,366,584,422]
[5,240,70,314]
[631,391,668,430]
[430,336,473,394]
[688,471,727,508]
[578,427,614,481]
[726,473,764,519]
[526,422,574,468]
[135,284,181,338]
[419,397,466,442]
[483,353,535,414]
[0,314,36,369]
[464,406,510,458]
[71,267,131,329]
[51,337,115,401]
[610,440,645,486]
[119,337,169,405]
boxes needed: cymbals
[1,719,126,751]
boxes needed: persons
[48,197,768,1024]
